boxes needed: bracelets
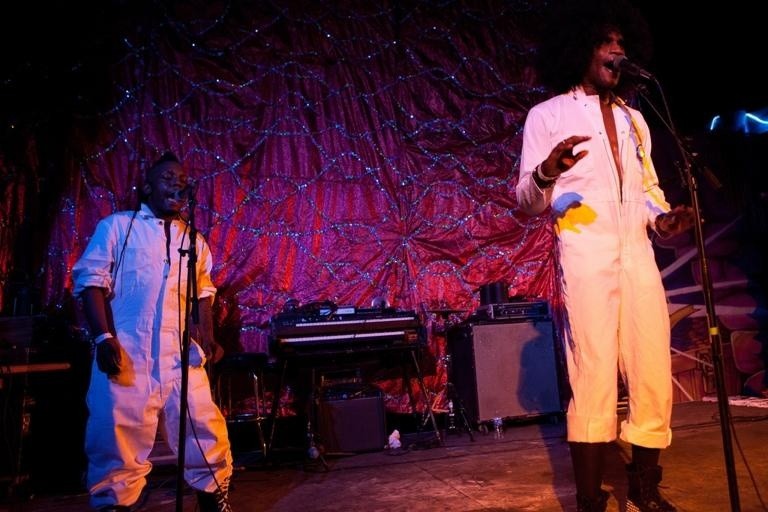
[532,162,560,189]
[94,331,115,345]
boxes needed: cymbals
[420,304,469,314]
[212,351,269,372]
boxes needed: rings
[563,140,566,144]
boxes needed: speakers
[446,317,562,424]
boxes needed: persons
[72,157,232,510]
[512,20,704,511]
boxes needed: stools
[214,352,270,457]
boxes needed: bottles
[492,410,504,441]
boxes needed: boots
[622,459,680,512]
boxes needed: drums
[318,382,373,400]
[226,412,272,466]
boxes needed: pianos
[268,305,428,358]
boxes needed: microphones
[612,54,655,82]
[174,182,195,202]
